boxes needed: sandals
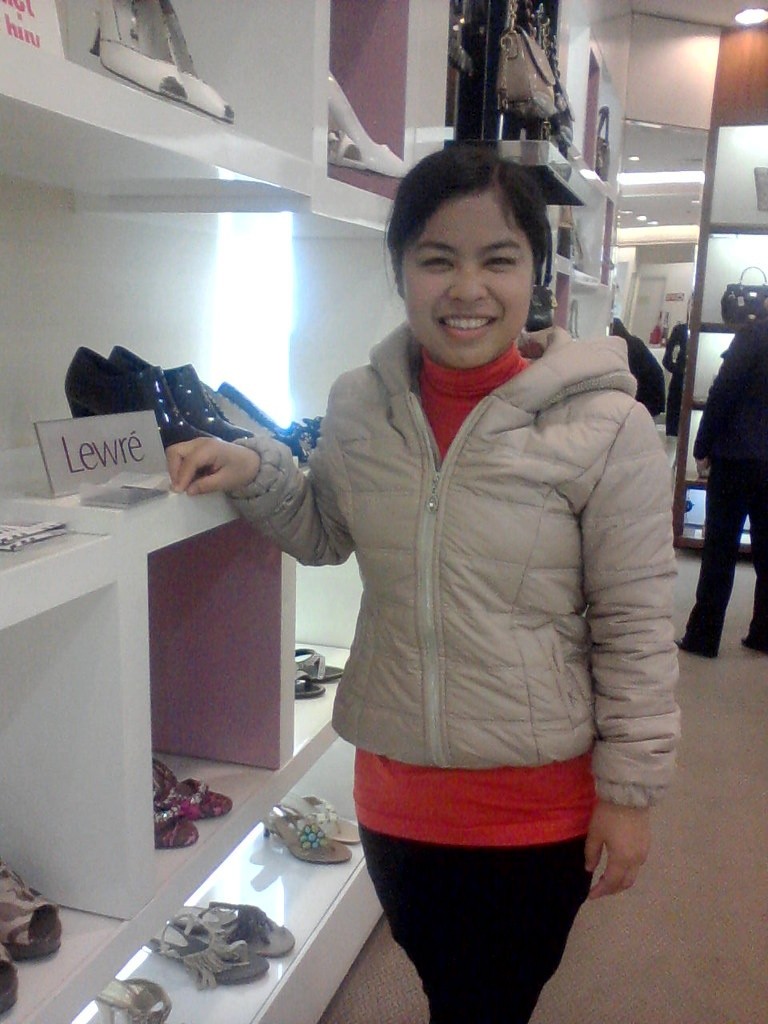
[295,649,344,682]
[153,802,199,848]
[296,681,326,699]
[152,757,232,817]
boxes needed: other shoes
[674,638,717,657]
[219,383,312,461]
[299,417,325,447]
[741,637,768,652]
[201,382,275,440]
[0,943,18,1013]
[0,857,62,959]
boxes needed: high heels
[64,346,225,450]
[94,978,173,1024]
[107,346,254,442]
[280,789,361,845]
[90,0,188,103]
[328,70,413,178]
[328,129,366,170]
[159,0,235,123]
[263,804,352,863]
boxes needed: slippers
[171,902,295,956]
[145,913,269,986]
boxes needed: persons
[165,141,684,1024]
[661,323,688,438]
[612,317,666,417]
[676,296,768,658]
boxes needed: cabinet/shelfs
[0,0,768,1024]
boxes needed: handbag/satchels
[596,108,608,180]
[497,1,558,121]
[721,267,768,323]
